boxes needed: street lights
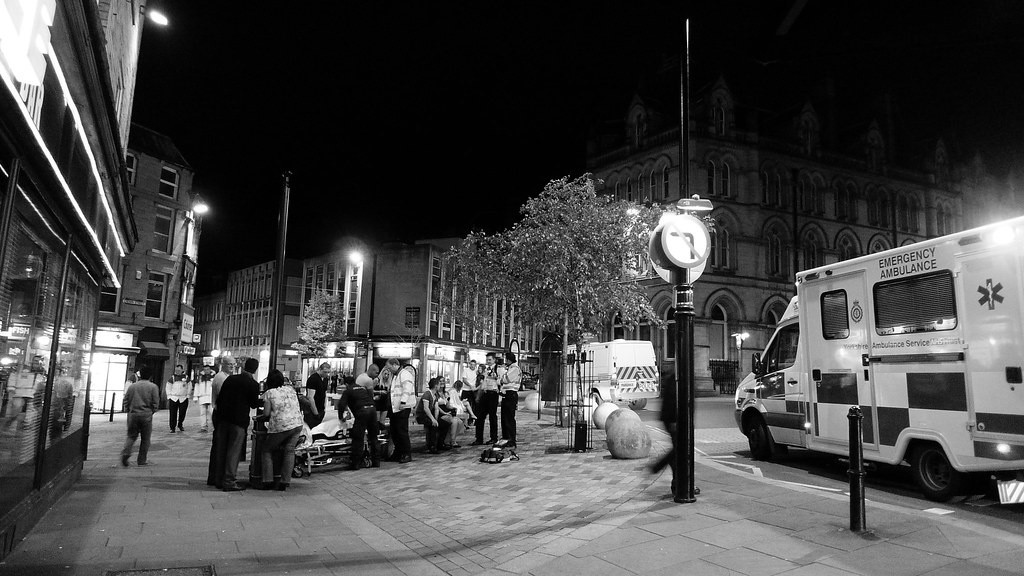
[672,197,714,502]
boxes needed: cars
[519,372,538,390]
[259,376,292,393]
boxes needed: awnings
[137,341,171,361]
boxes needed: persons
[165,365,192,433]
[468,352,522,445]
[416,376,477,453]
[461,360,486,426]
[302,362,339,467]
[121,366,159,466]
[207,356,304,491]
[339,358,416,469]
[193,364,216,433]
[0,355,74,446]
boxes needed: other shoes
[346,462,360,470]
[201,428,206,432]
[170,428,175,433]
[486,438,498,444]
[178,425,184,431]
[372,460,380,467]
[122,454,131,466]
[279,482,289,491]
[443,441,461,448]
[426,444,438,454]
[138,462,149,466]
[472,439,483,445]
[384,452,412,463]
[63,426,69,431]
[502,440,516,448]
[207,476,246,491]
[437,442,451,450]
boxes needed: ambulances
[735,215,1024,504]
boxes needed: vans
[535,339,660,410]
